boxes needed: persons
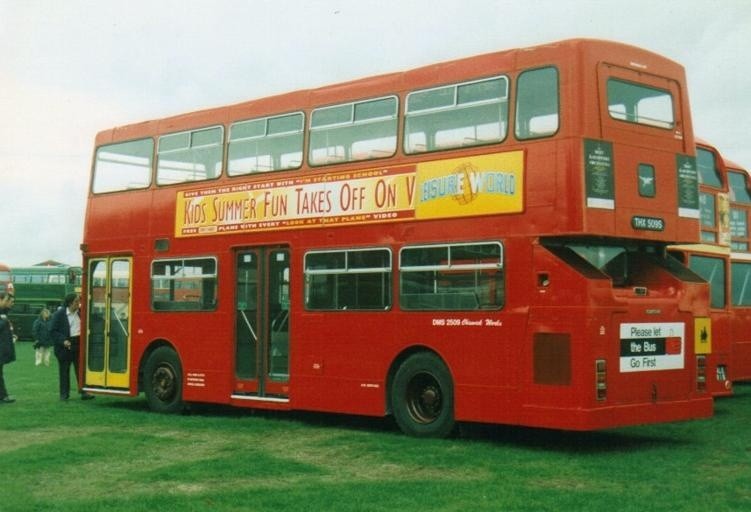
[47,293,95,401]
[0,292,17,403]
[31,308,54,368]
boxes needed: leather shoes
[0,397,16,403]
[81,393,96,400]
[60,394,69,400]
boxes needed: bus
[90,271,203,302]
[694,136,735,398]
[0,264,84,339]
[720,153,751,390]
[78,37,717,442]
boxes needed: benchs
[123,134,494,190]
